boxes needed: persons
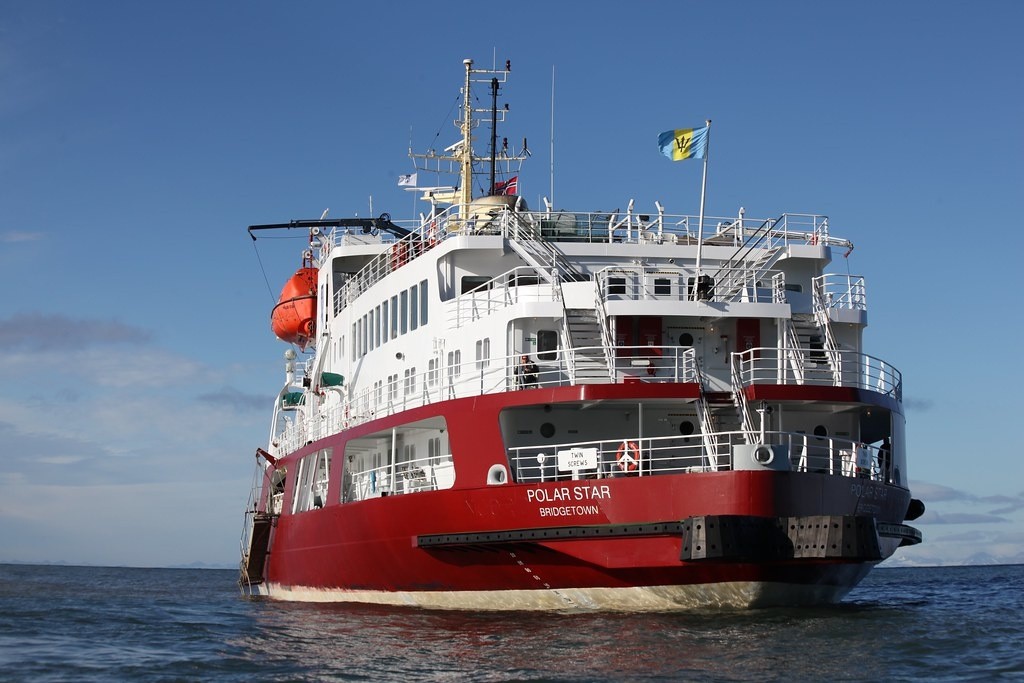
[877,436,892,484]
[514,355,539,391]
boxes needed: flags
[657,126,710,161]
[488,176,518,195]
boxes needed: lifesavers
[616,441,639,471]
[428,221,437,248]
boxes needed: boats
[241,45,924,619]
